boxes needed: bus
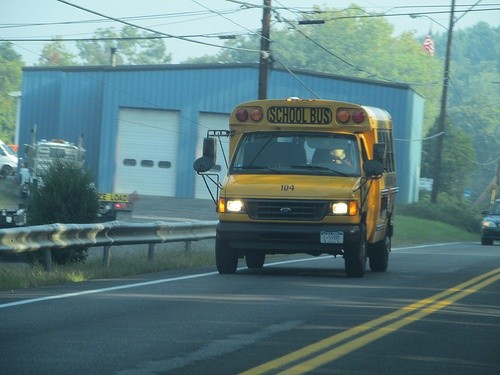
[191,95,398,279]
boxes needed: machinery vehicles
[18,121,138,222]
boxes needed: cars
[481,199,500,246]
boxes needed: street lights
[408,0,480,202]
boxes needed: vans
[0,140,19,178]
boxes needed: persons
[328,147,352,168]
